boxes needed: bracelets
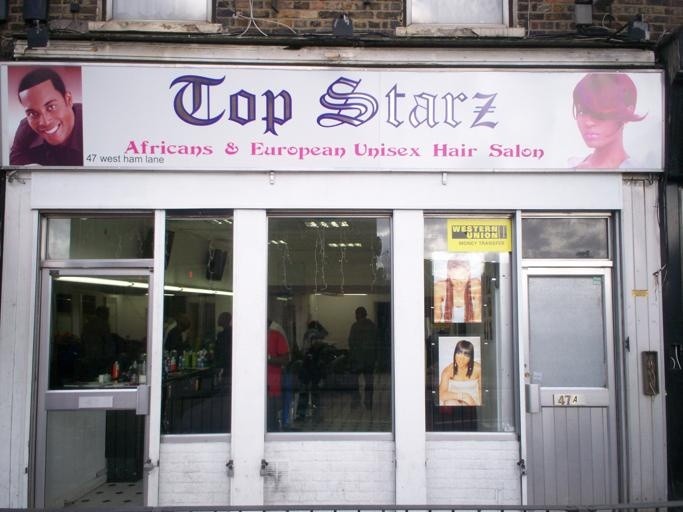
[266,352,270,362]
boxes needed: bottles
[111,344,215,386]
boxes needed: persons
[212,311,230,379]
[266,316,290,428]
[163,316,194,349]
[438,340,480,407]
[434,255,481,323]
[570,75,647,169]
[94,306,120,341]
[264,319,289,431]
[293,320,328,423]
[8,67,83,163]
[348,306,375,410]
